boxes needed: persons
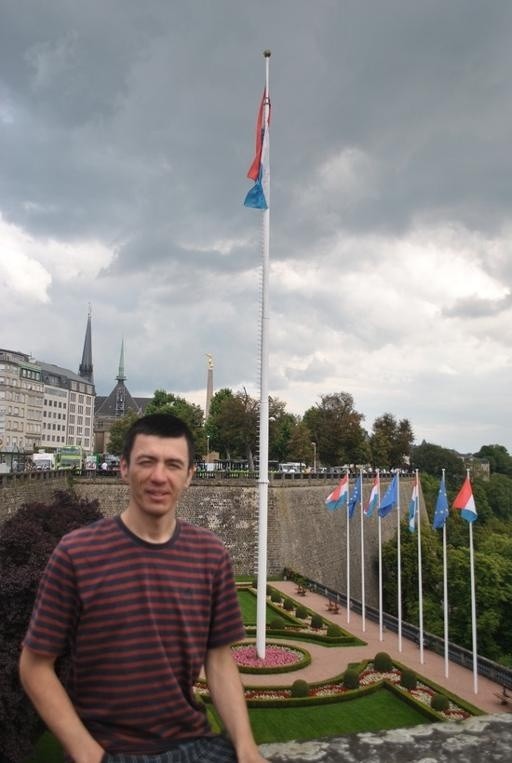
[19,413,269,763]
[100,460,108,470]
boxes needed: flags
[409,484,418,532]
[347,479,361,517]
[325,474,347,512]
[243,87,271,210]
[452,476,478,522]
[432,480,450,530]
[378,475,397,518]
[363,479,378,518]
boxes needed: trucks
[32,453,55,470]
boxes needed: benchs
[295,583,306,596]
[494,680,512,708]
[326,598,340,614]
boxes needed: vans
[279,463,306,473]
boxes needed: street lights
[312,441,316,472]
[206,435,209,463]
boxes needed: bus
[54,445,85,474]
